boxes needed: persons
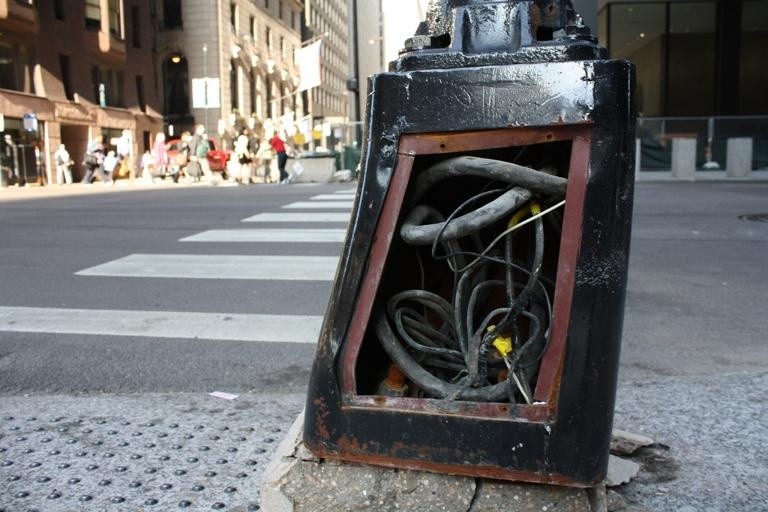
[0,124,293,187]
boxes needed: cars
[164,138,231,179]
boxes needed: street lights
[201,43,209,136]
